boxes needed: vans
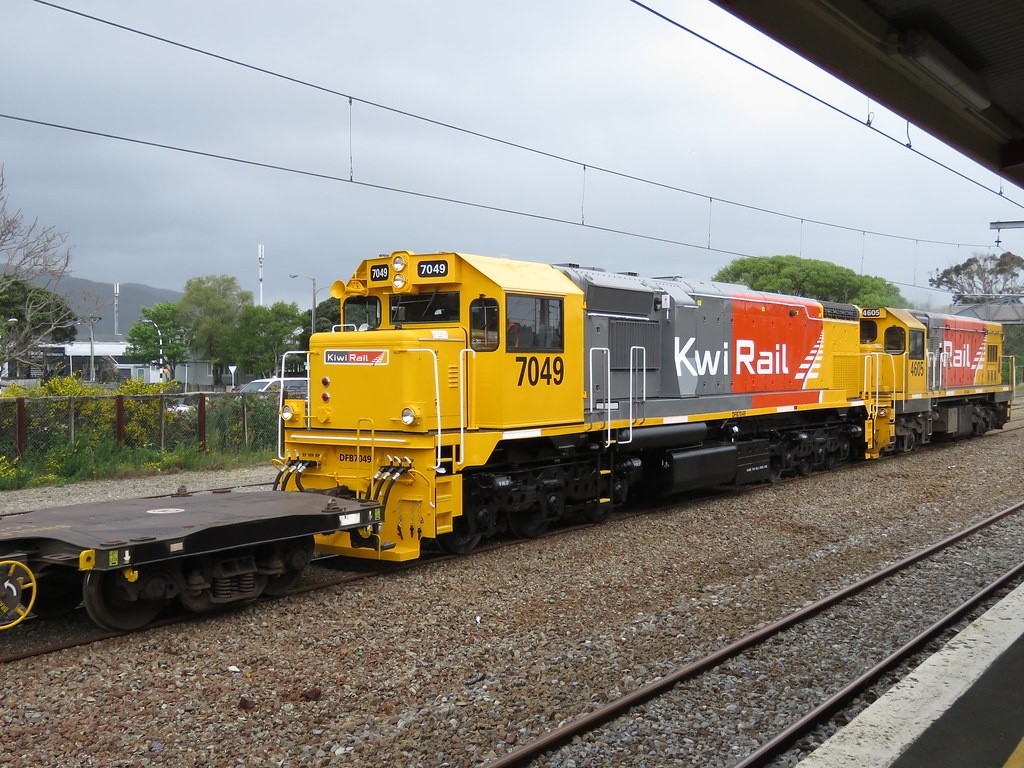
[235,377,307,402]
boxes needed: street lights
[143,318,165,393]
[290,272,317,335]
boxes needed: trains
[266,251,1016,564]
